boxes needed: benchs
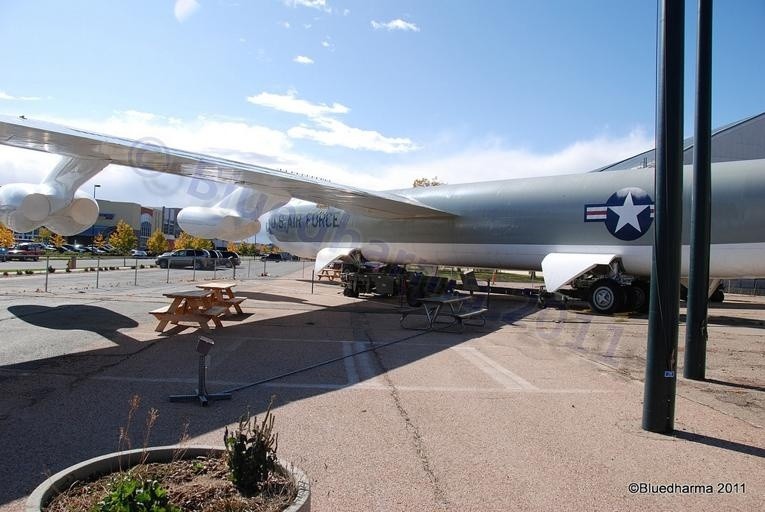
[399,305,489,334]
[317,274,340,281]
[148,294,247,334]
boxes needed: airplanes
[0,109,765,316]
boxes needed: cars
[345,262,418,288]
[0,239,241,269]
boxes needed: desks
[318,269,341,281]
[154,282,243,334]
[416,295,473,332]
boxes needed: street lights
[92,184,101,256]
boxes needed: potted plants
[20,391,312,512]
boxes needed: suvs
[260,253,283,263]
